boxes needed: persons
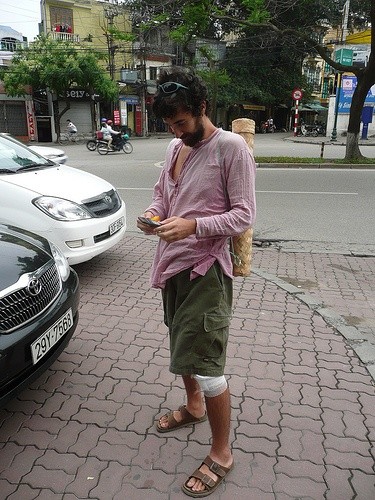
[314,114,318,123]
[138,73,257,496]
[300,118,306,125]
[95,118,122,149]
[65,119,78,142]
[267,117,274,129]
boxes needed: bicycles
[261,124,276,134]
[58,129,85,145]
[298,120,326,137]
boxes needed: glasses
[157,81,190,93]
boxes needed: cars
[1,133,127,265]
[0,224,80,405]
[27,146,68,164]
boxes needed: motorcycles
[87,137,97,151]
[96,132,133,155]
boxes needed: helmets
[107,119,113,124]
[101,117,107,122]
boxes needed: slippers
[181,454,235,498]
[155,404,209,433]
